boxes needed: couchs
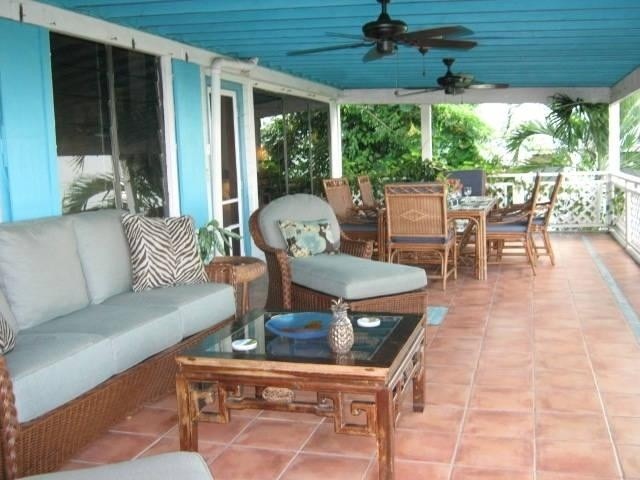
[0,207,236,480]
[16,452,214,480]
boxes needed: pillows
[277,219,337,257]
[123,213,208,292]
[0,313,15,354]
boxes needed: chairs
[358,176,385,211]
[387,170,561,293]
[249,194,427,314]
[321,178,386,261]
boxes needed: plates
[264,311,334,341]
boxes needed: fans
[394,56,510,98]
[286,0,478,64]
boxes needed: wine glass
[447,187,472,209]
